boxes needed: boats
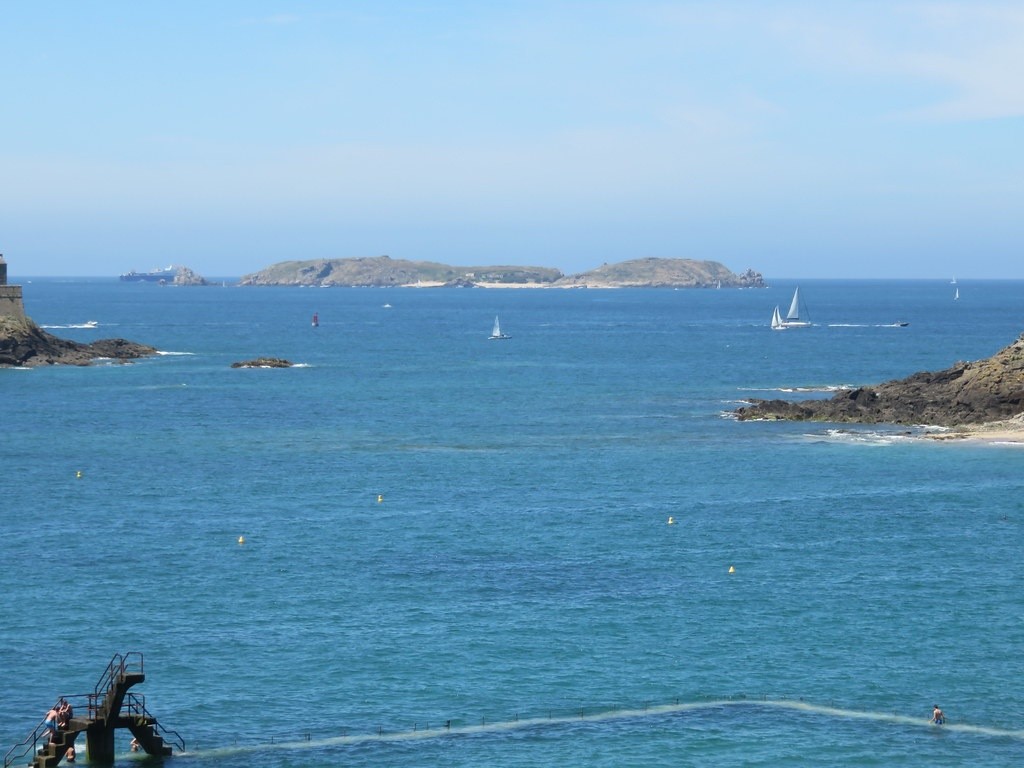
[894,322,910,326]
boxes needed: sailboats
[770,305,790,330]
[488,313,511,340]
[781,284,811,328]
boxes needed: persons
[129,738,139,752]
[44,706,62,743]
[64,745,76,762]
[929,704,945,724]
[58,699,73,726]
